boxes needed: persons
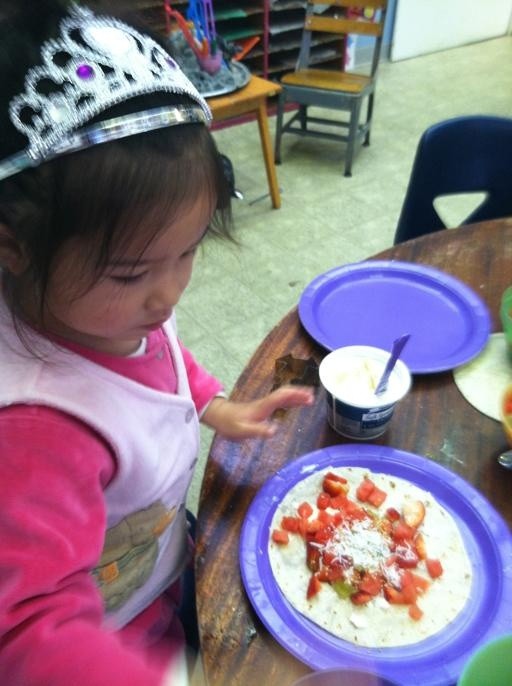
[0,0,316,686]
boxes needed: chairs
[393,116,511,243]
[275,1,389,177]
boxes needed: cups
[319,346,411,442]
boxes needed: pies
[267,464,473,649]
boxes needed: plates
[239,442,512,686]
[297,261,490,372]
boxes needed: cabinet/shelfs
[103,1,351,132]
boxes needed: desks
[201,74,284,210]
